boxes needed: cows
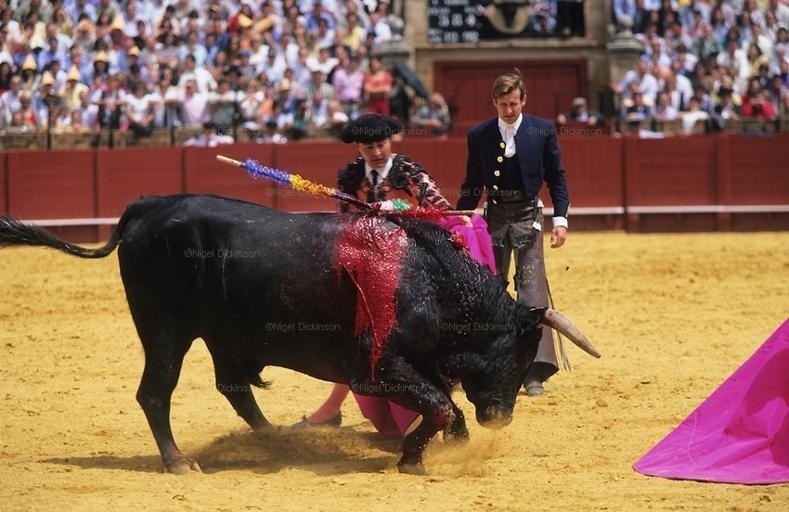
[0,193,603,477]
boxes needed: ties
[370,167,381,184]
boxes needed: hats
[339,113,402,146]
[23,16,140,87]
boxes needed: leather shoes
[526,380,544,396]
[290,411,342,429]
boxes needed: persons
[293,114,456,428]
[456,75,570,395]
